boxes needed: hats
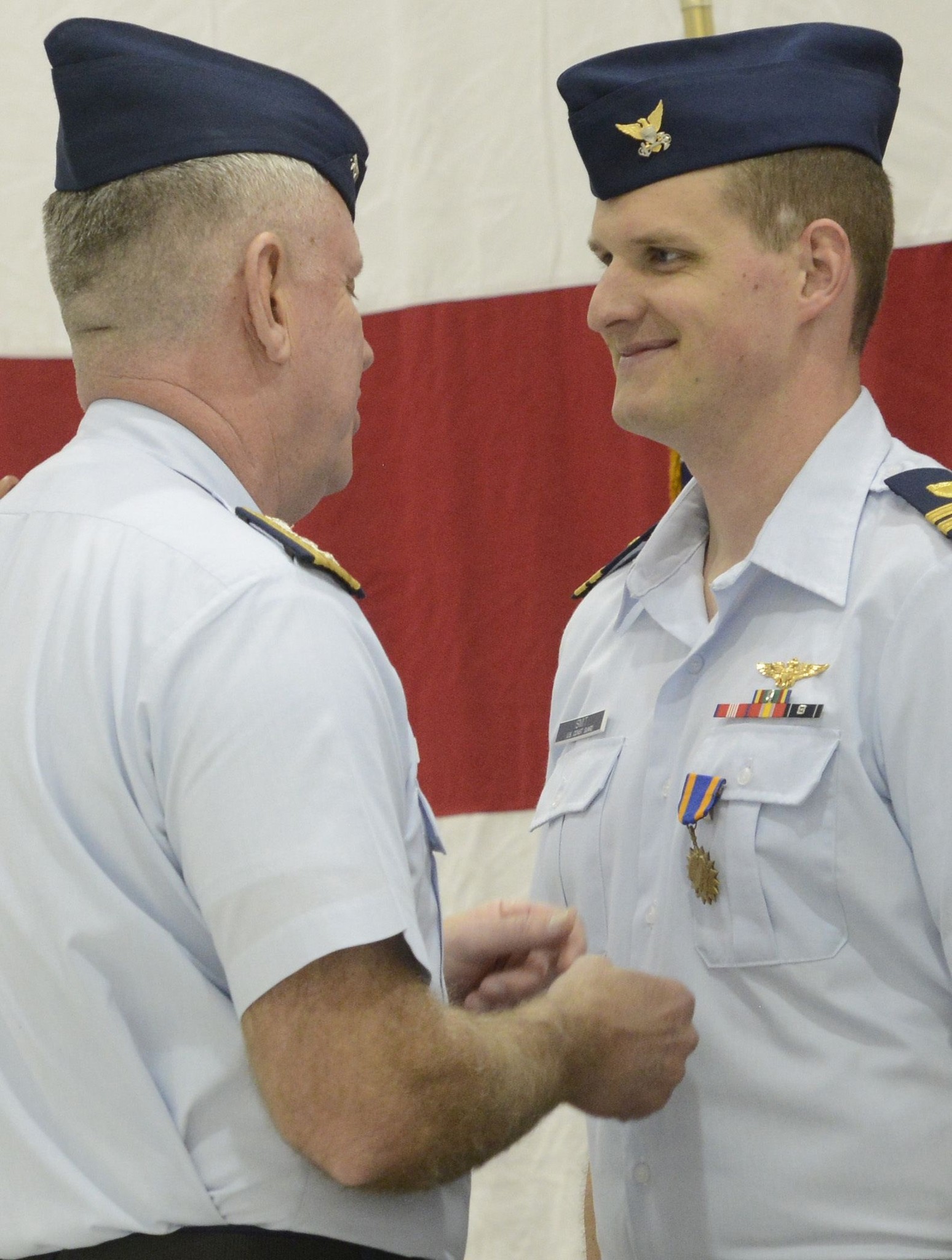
[42,17,370,222]
[555,21,903,201]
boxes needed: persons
[0,17,697,1260]
[526,21,952,1260]
